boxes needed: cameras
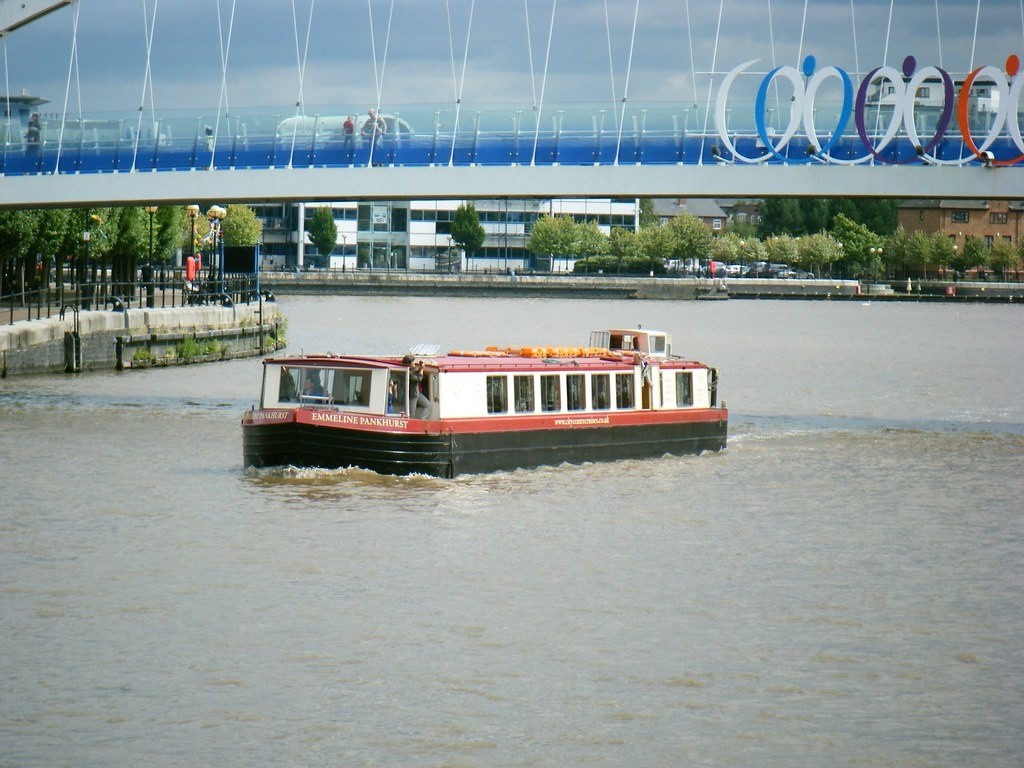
[419,361,423,365]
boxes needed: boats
[241,324,727,478]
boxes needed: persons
[295,376,325,404]
[28,113,41,141]
[361,109,387,167]
[343,116,353,147]
[402,355,432,419]
[388,380,397,414]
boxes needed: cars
[664,259,814,279]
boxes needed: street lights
[186,203,201,309]
[953,244,958,282]
[834,241,844,279]
[447,235,453,274]
[145,205,159,308]
[206,204,227,307]
[341,233,348,274]
[739,240,745,276]
[870,246,883,284]
